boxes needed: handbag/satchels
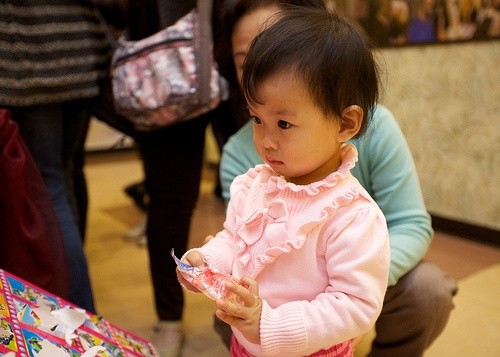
[112,10,221,129]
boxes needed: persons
[176,8,390,357]
[89,0,250,357]
[209,0,460,357]
[0,0,106,315]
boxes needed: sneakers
[152,321,183,357]
